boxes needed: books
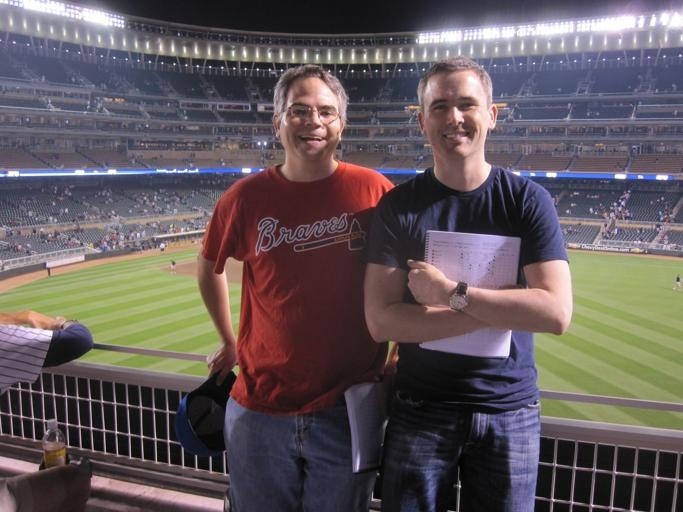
[415,230,523,361]
[340,378,390,474]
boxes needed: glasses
[283,105,340,125]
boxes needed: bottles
[41,418,66,468]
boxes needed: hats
[174,371,237,457]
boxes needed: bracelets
[53,316,75,330]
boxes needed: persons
[194,64,398,510]
[671,274,681,291]
[0,308,96,403]
[45,258,51,276]
[361,59,572,512]
[1,146,683,273]
[169,258,176,277]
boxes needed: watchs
[448,279,472,318]
